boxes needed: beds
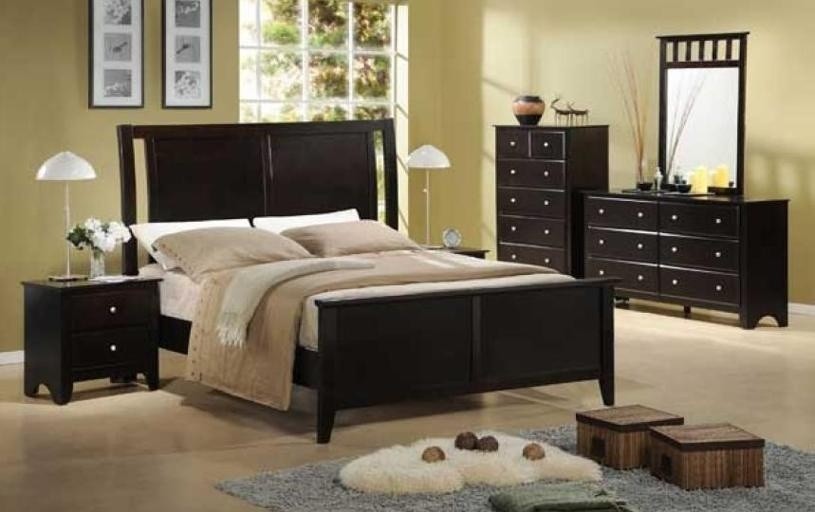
[107,114,640,448]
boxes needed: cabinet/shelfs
[485,115,613,284]
[571,182,795,333]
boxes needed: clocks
[438,223,465,249]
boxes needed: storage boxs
[639,414,769,495]
[569,401,688,474]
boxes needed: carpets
[192,399,815,511]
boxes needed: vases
[86,243,106,280]
[508,91,547,131]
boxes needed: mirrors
[648,26,753,197]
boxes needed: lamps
[402,142,456,250]
[31,147,100,284]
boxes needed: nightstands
[436,243,491,265]
[18,266,166,405]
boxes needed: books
[88,276,139,285]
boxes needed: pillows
[272,216,426,260]
[146,224,319,288]
[248,205,363,239]
[125,213,256,274]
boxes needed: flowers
[55,213,136,265]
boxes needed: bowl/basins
[637,181,693,193]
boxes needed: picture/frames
[84,0,146,110]
[158,0,214,110]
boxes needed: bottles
[511,95,546,126]
